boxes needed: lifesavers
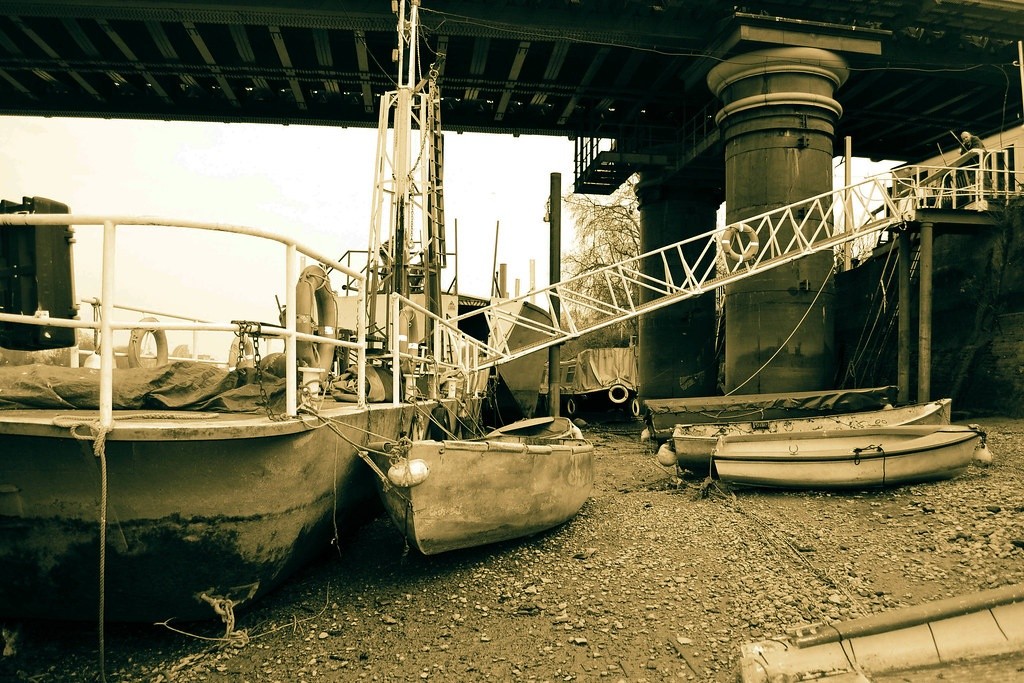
[431,407,452,441]
[608,383,630,405]
[128,318,169,367]
[565,397,580,416]
[229,335,254,369]
[628,398,640,417]
[296,264,339,377]
[399,306,419,374]
[723,223,759,260]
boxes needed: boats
[640,384,985,491]
[541,345,639,422]
[1,1,497,654]
[363,414,596,558]
[283,216,551,429]
[736,580,1024,682]
